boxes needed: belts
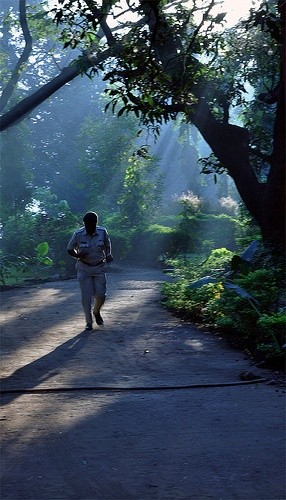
[80,259,105,267]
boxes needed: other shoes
[94,312,103,325]
[85,323,93,331]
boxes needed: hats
[83,212,98,223]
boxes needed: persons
[67,212,113,330]
